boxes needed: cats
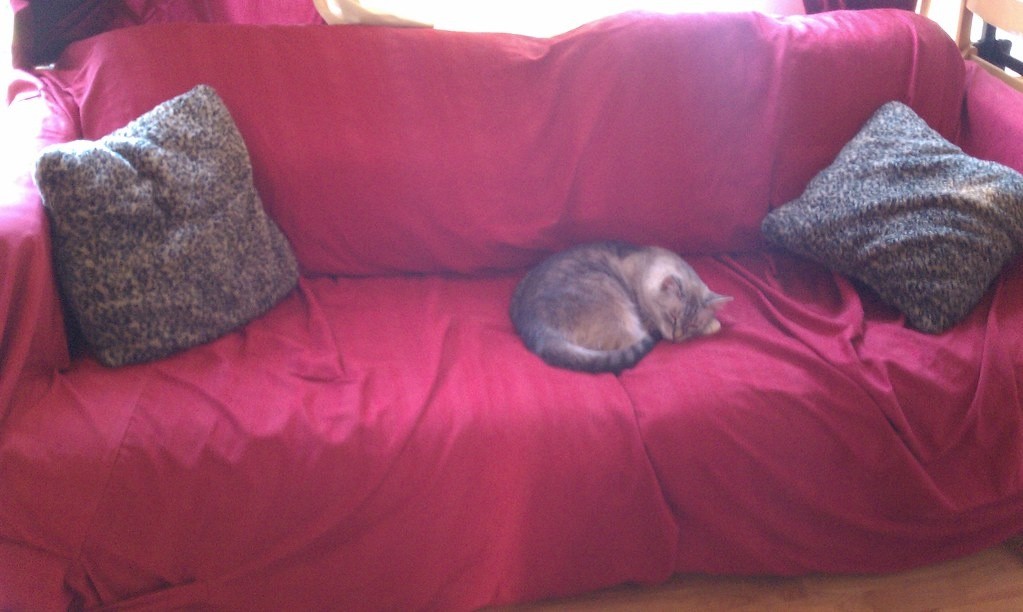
[510,240,734,374]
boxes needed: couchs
[0,10,1023,611]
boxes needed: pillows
[759,100,1023,334]
[34,86,298,365]
